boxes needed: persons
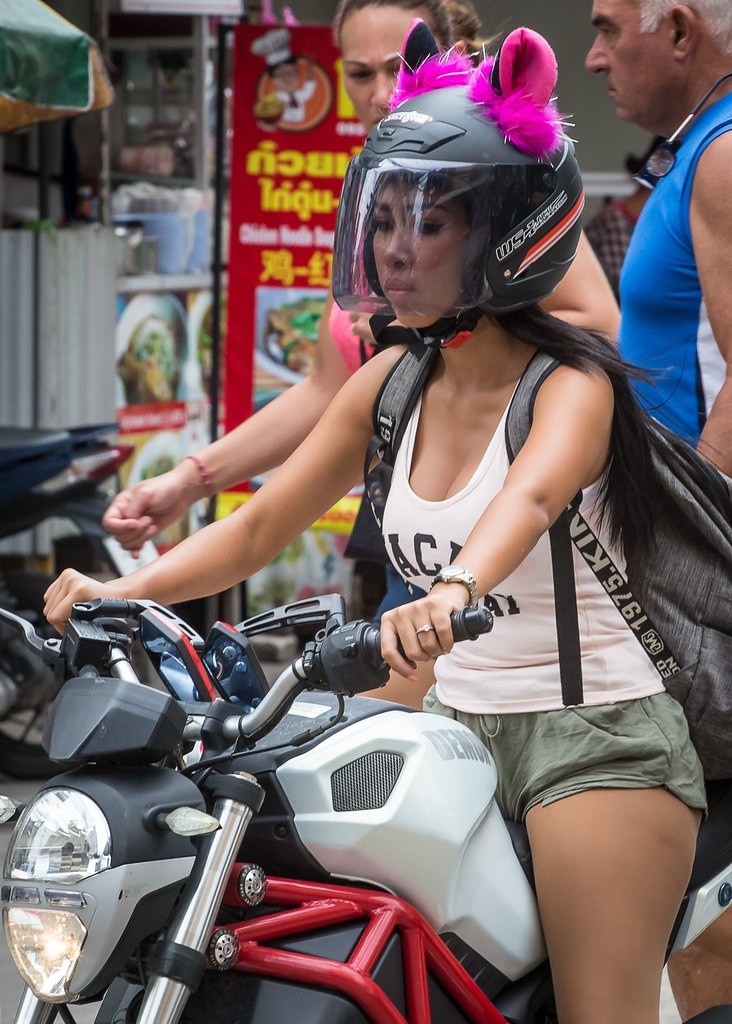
[102,0,621,559]
[586,0,732,1024]
[43,20,707,1024]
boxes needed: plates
[115,293,216,555]
[252,291,325,384]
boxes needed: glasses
[624,72,730,190]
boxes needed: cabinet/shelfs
[0,0,245,227]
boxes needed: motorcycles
[0,584,732,1024]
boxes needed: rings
[416,625,436,633]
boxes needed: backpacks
[344,336,731,790]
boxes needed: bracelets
[188,456,215,500]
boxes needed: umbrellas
[0,0,117,134]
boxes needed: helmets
[330,85,587,315]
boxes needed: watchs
[430,564,479,608]
